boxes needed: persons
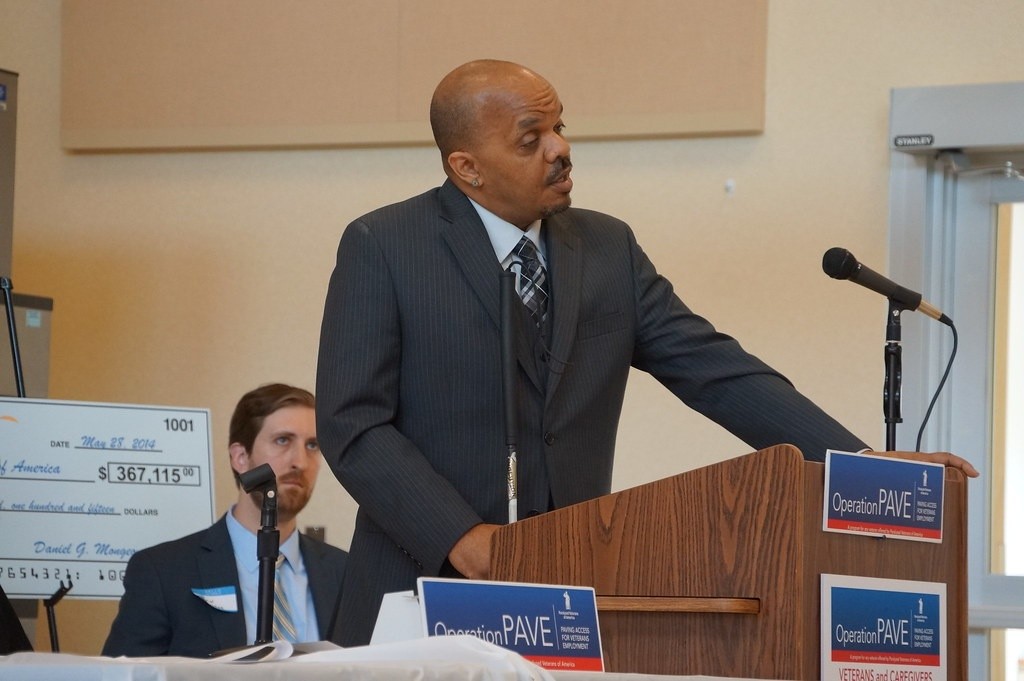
[318,63,979,645]
[101,382,348,657]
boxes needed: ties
[512,236,551,330]
[270,552,298,650]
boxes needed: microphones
[822,246,954,326]
